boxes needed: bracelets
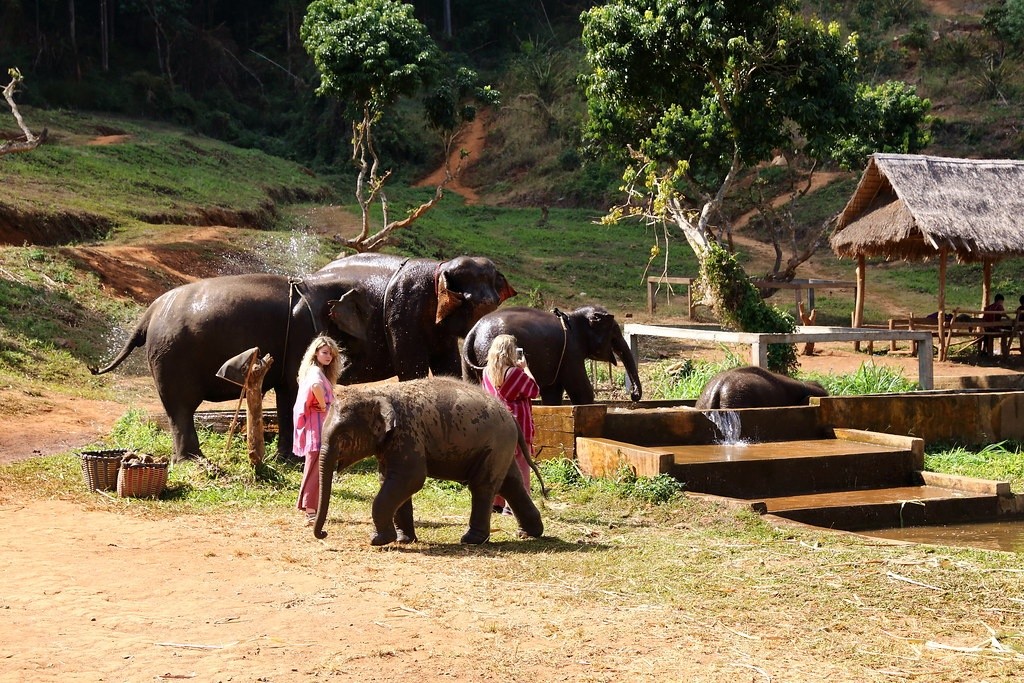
[322,405,327,409]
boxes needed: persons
[292,336,343,517]
[982,294,1012,357]
[482,334,539,516]
[1016,294,1024,354]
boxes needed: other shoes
[302,514,317,527]
[503,507,513,515]
[492,506,502,513]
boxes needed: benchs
[862,323,940,354]
[932,331,1023,362]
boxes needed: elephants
[695,364,827,412]
[86,252,642,478]
[312,376,544,546]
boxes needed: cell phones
[516,348,523,362]
